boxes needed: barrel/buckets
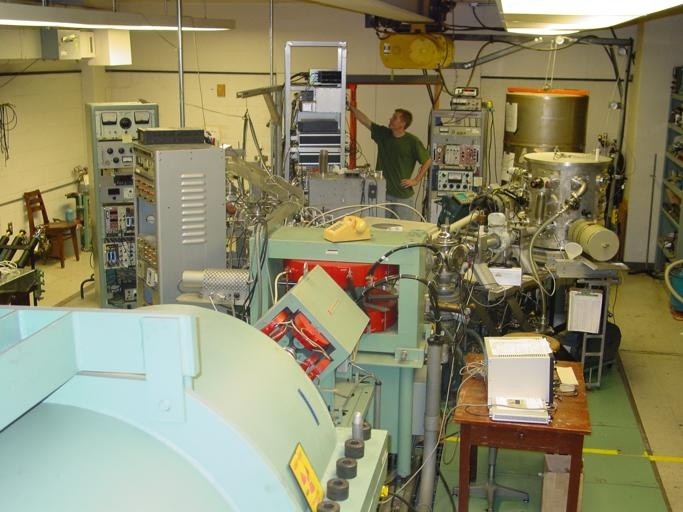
[670,268,683,312]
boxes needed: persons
[346,98,432,221]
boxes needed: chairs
[23,189,80,269]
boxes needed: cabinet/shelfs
[650,65,682,279]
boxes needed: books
[489,266,522,286]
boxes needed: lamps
[0,0,235,32]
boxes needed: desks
[450,351,594,512]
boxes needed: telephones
[324,215,371,243]
